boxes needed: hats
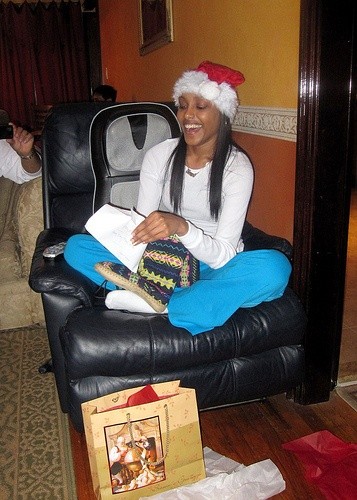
[171,60,245,125]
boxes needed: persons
[63,61,292,336]
[0,122,42,184]
[93,85,117,102]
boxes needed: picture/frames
[138,0,173,55]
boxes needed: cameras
[0,125,13,139]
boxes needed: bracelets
[21,152,35,160]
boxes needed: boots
[95,231,200,313]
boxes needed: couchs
[26,101,314,433]
[0,175,44,333]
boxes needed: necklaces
[186,169,199,177]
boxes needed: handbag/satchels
[80,379,206,500]
